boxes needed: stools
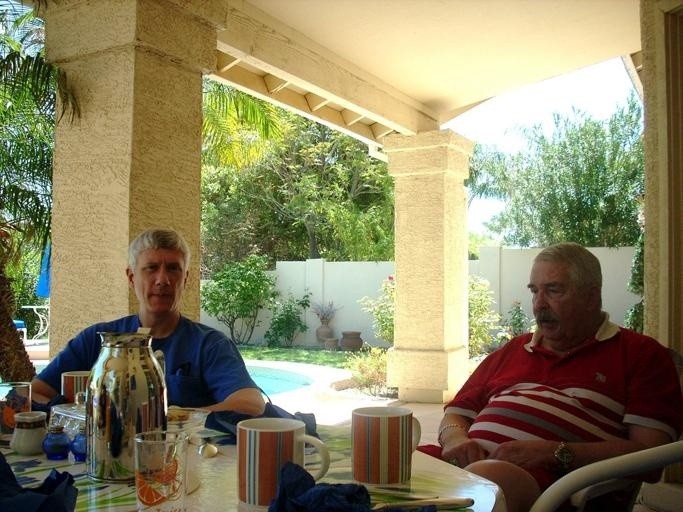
[11,320,29,345]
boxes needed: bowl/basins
[164,409,210,438]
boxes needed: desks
[20,305,50,340]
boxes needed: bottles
[42,425,86,463]
[9,410,48,456]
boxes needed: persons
[437,242,680,512]
[31,227,266,418]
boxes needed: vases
[316,320,333,343]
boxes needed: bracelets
[438,424,468,448]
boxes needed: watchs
[554,441,574,472]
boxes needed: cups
[352,404,421,485]
[61,370,92,402]
[132,431,188,512]
[235,417,329,509]
[0,382,33,449]
